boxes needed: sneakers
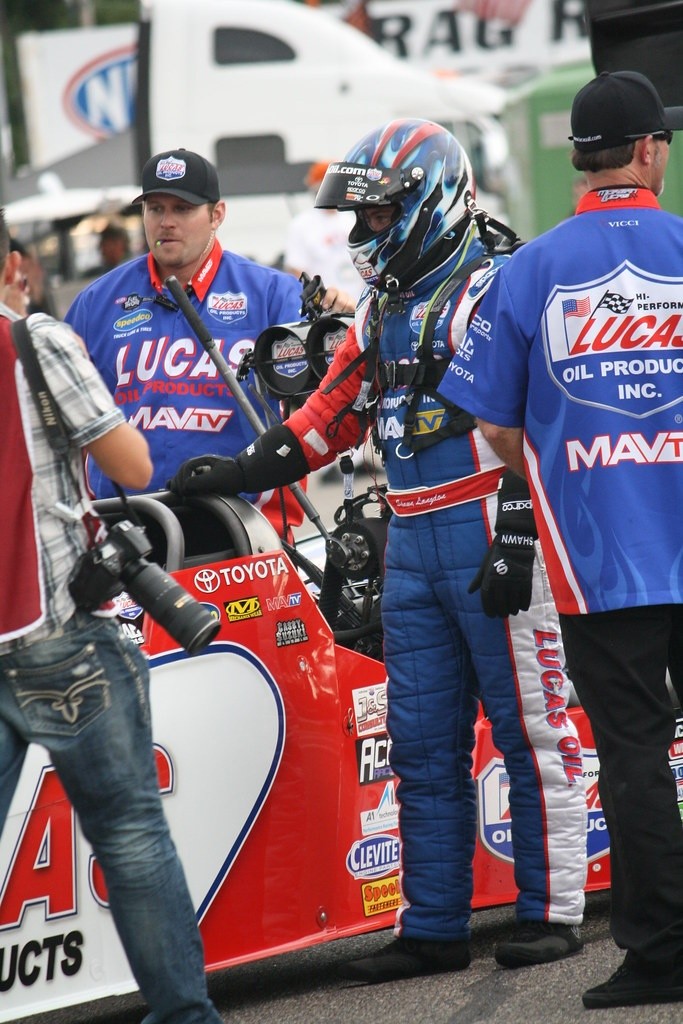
[494,922,584,968]
[334,938,472,980]
[584,953,681,1006]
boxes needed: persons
[434,71,683,1008]
[0,202,229,1024]
[61,147,362,566]
[167,113,593,983]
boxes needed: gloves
[165,455,244,499]
[466,531,535,619]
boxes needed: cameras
[68,521,222,655]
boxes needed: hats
[132,149,221,207]
[569,71,683,152]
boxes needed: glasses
[11,274,31,293]
[625,129,674,146]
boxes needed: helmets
[313,119,474,288]
